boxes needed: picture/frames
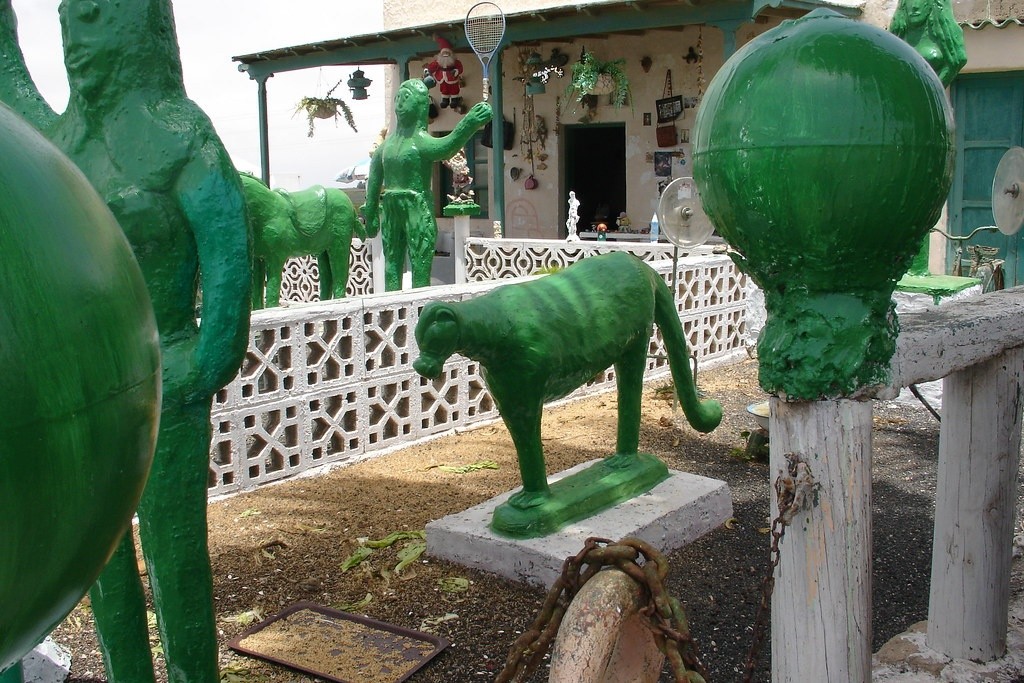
[655,95,684,124]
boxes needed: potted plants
[559,50,636,117]
[291,79,357,137]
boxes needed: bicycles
[930,224,1005,295]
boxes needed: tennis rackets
[464,1,507,103]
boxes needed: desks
[579,231,724,243]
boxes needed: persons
[423,35,463,109]
[364,78,495,293]
[889,0,970,279]
[1,0,259,683]
[441,146,475,203]
